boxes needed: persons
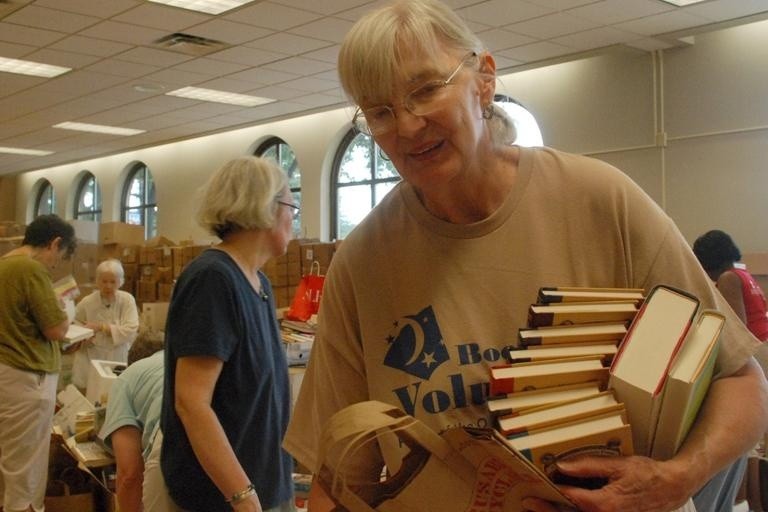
[159,153,298,512]
[71,254,142,401]
[97,327,183,510]
[281,0,768,512]
[0,212,92,512]
[692,227,768,347]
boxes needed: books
[276,313,319,370]
[52,273,83,303]
[51,381,116,473]
[484,281,726,492]
[289,472,315,512]
[62,318,94,353]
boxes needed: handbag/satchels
[327,423,582,512]
[43,478,96,512]
[286,260,328,321]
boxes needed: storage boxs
[0,221,345,331]
[104,495,114,512]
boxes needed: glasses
[352,52,476,138]
[276,200,300,219]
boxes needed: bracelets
[224,483,255,504]
[230,489,256,507]
